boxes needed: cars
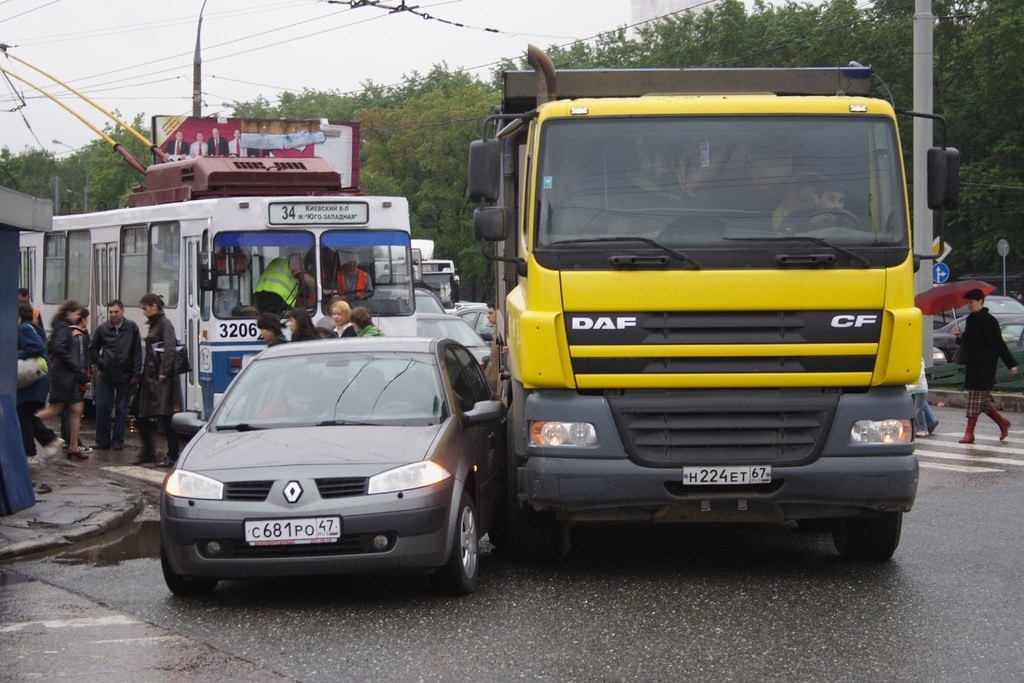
[934,312,1024,361]
[414,314,492,364]
[155,334,506,597]
[934,293,1024,331]
[449,303,498,337]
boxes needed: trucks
[466,44,961,562]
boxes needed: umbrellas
[915,280,997,330]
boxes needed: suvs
[367,286,447,338]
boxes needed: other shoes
[153,456,177,468]
[89,441,112,450]
[110,442,123,450]
[128,453,157,464]
[28,457,41,465]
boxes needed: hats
[257,313,288,330]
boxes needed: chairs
[771,171,828,231]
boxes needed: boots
[959,417,978,443]
[983,402,1012,441]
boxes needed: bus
[366,236,462,311]
[18,154,424,438]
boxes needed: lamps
[216,111,227,124]
[306,118,329,126]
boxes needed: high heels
[66,448,89,460]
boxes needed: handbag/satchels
[16,355,49,390]
[152,338,193,380]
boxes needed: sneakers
[41,437,65,460]
[77,443,93,453]
[916,429,929,437]
[62,441,70,451]
[927,421,939,434]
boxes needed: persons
[165,127,272,158]
[955,289,1018,443]
[906,356,938,437]
[776,177,859,234]
[256,300,384,348]
[18,288,186,466]
[254,247,374,313]
[480,302,499,395]
